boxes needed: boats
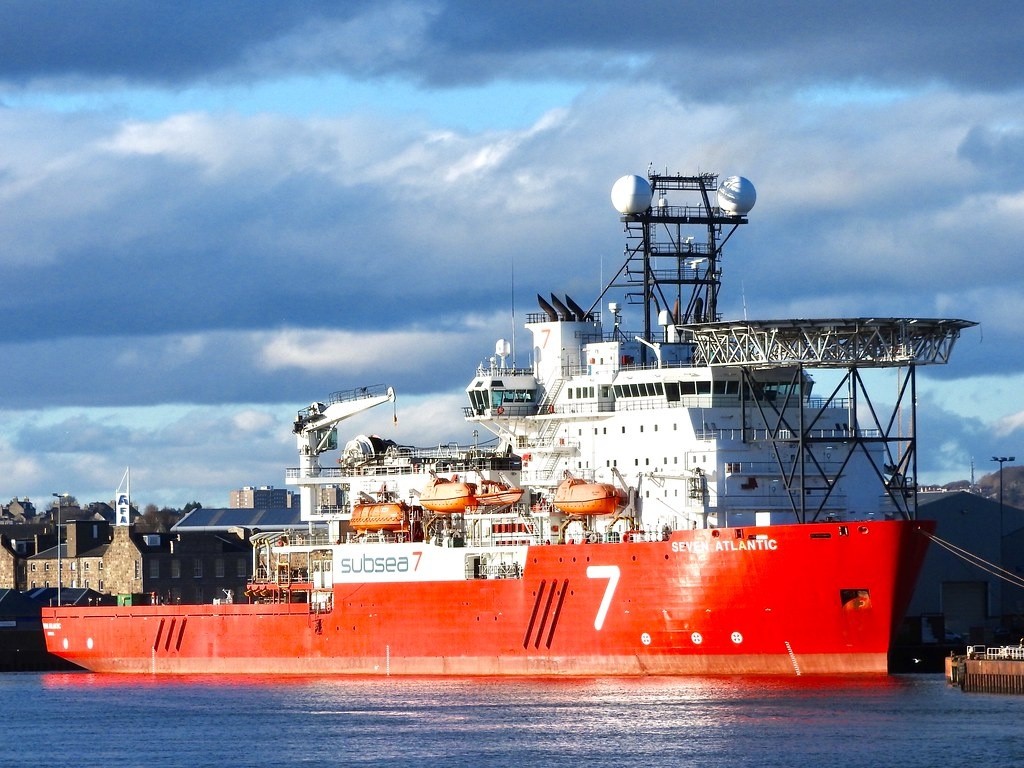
[38,167,983,677]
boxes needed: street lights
[52,492,69,606]
